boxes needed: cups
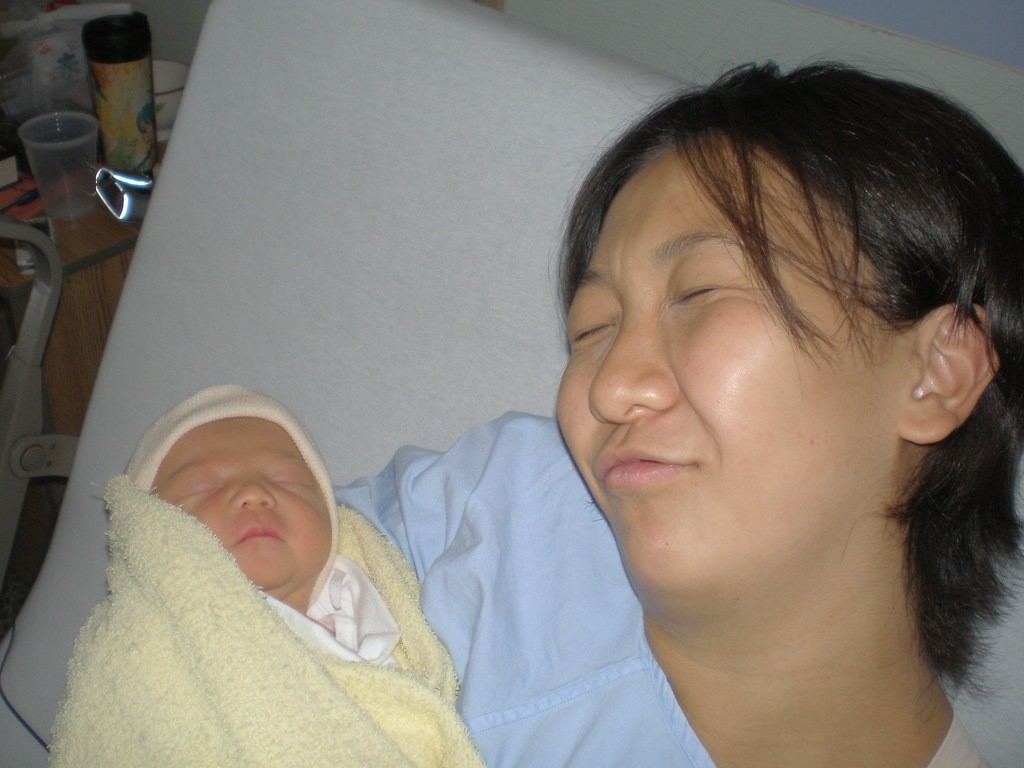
[18,111,99,223]
[81,12,156,174]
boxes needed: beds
[1,1,1024,768]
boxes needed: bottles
[0,136,18,188]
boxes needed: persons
[51,382,488,768]
[331,59,1022,768]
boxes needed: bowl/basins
[154,60,190,142]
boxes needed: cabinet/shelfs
[1,137,168,555]
